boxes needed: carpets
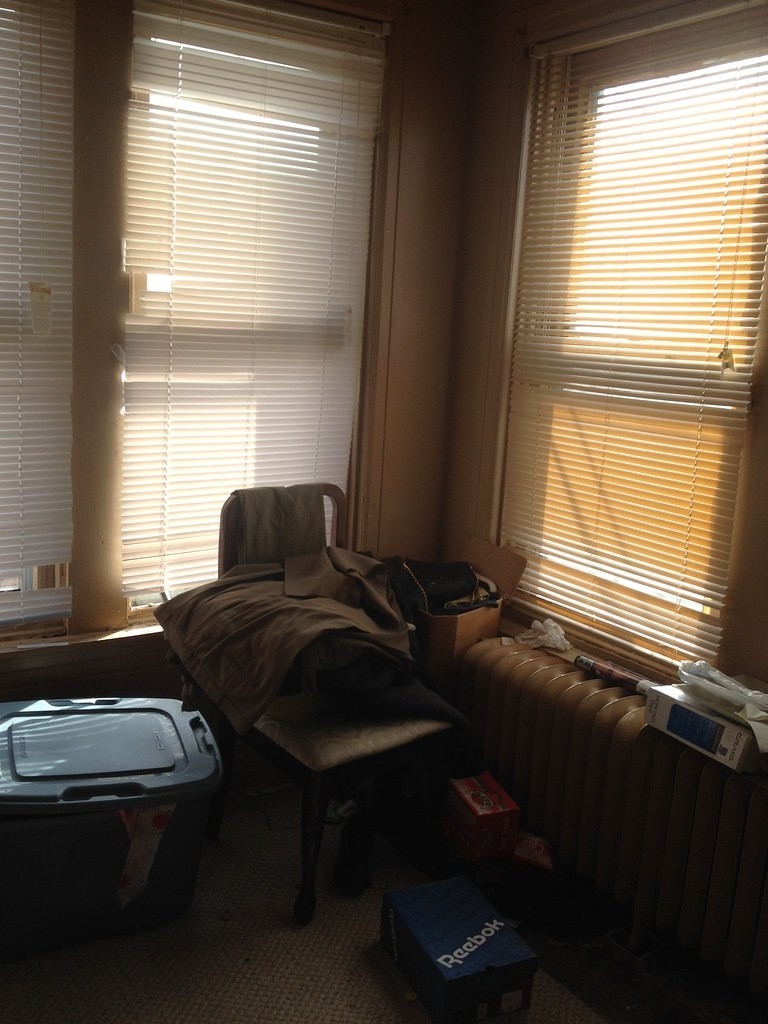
[378,802,768,1024]
[1,779,604,1023]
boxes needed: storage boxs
[644,684,766,774]
[0,698,223,963]
[438,767,517,863]
[380,876,538,1024]
[404,538,528,696]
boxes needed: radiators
[456,635,768,1003]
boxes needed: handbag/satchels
[400,556,479,614]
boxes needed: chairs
[213,483,454,925]
